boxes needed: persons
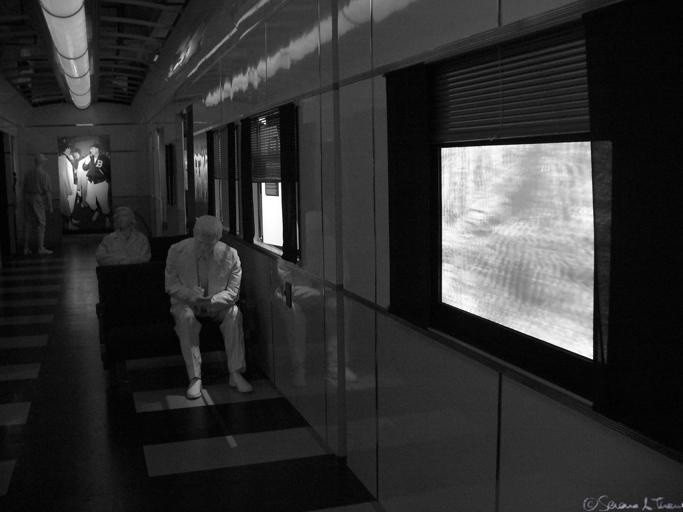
[162,215,254,400]
[21,152,56,257]
[94,205,154,267]
[56,143,109,235]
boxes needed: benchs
[95,262,251,383]
